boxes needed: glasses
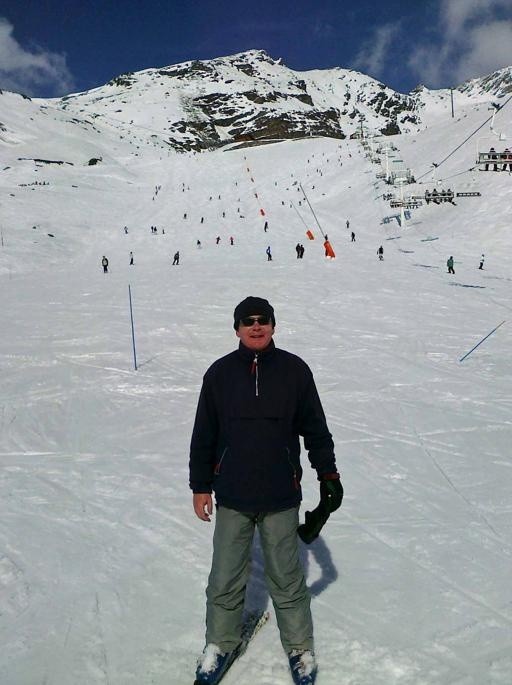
[240,315,270,327]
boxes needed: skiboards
[191,610,319,685]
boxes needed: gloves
[295,493,336,544]
[314,463,345,512]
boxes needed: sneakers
[288,648,320,685]
[194,642,227,685]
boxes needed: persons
[446,255,455,274]
[376,246,384,260]
[484,147,498,172]
[296,244,300,258]
[171,251,180,265]
[266,246,272,261]
[300,245,304,258]
[424,189,453,204]
[130,251,134,264]
[187,294,342,685]
[478,253,484,270]
[498,148,511,171]
[102,256,109,273]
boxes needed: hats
[233,295,276,324]
